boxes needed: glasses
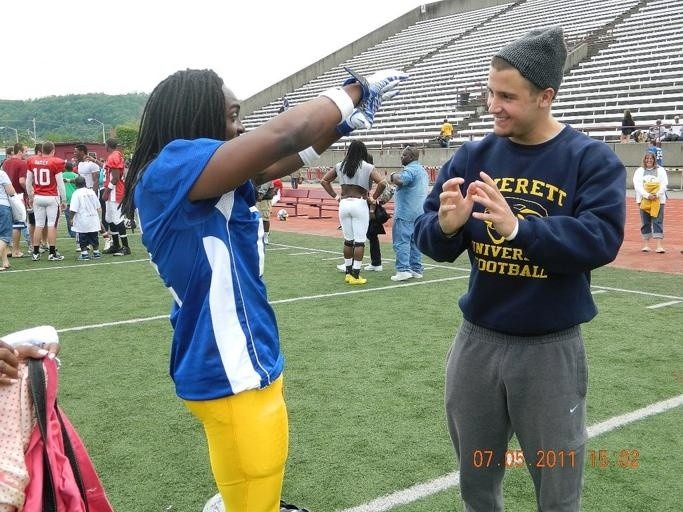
[406,146,414,155]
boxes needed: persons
[0,324,61,384]
[254,167,305,245]
[633,151,668,253]
[386,145,429,281]
[620,110,683,144]
[336,152,393,272]
[0,138,136,271]
[437,118,454,148]
[278,96,289,115]
[411,26,625,511]
[320,140,387,285]
[114,69,411,512]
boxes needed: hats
[493,25,567,100]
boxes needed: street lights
[0,126,18,143]
[87,117,106,144]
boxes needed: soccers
[277,209,288,221]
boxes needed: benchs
[308,190,341,218]
[272,189,307,218]
[370,192,393,219]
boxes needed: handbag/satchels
[374,204,392,223]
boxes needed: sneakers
[0,237,131,271]
[641,247,652,251]
[654,247,665,253]
[337,263,423,285]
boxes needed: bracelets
[370,197,374,201]
[334,194,340,201]
[390,172,395,182]
[297,146,321,168]
[319,85,354,127]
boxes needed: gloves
[336,79,401,136]
[343,66,409,108]
